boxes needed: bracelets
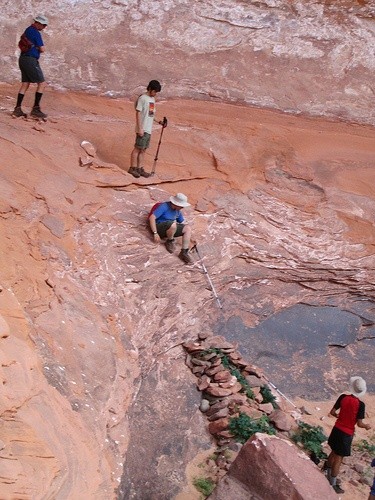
[153,232,157,234]
[159,121,162,124]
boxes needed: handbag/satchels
[18,33,35,53]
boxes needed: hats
[33,15,49,25]
[169,193,191,208]
[349,376,367,398]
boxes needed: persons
[149,193,193,264]
[368,458,375,500]
[327,376,372,494]
[13,15,48,118]
[127,80,167,178]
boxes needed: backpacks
[148,201,169,220]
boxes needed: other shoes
[12,106,27,118]
[31,106,48,118]
[164,239,175,254]
[178,252,194,264]
[128,167,140,178]
[136,167,151,177]
[333,485,344,494]
